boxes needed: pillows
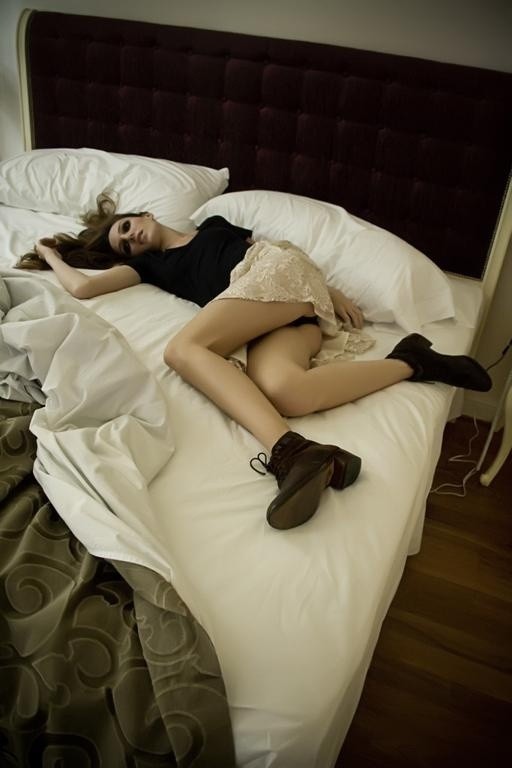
[188,184,458,339]
[1,142,234,240]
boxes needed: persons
[11,190,492,530]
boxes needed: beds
[2,4,510,767]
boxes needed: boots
[386,332,492,395]
[250,431,362,531]
